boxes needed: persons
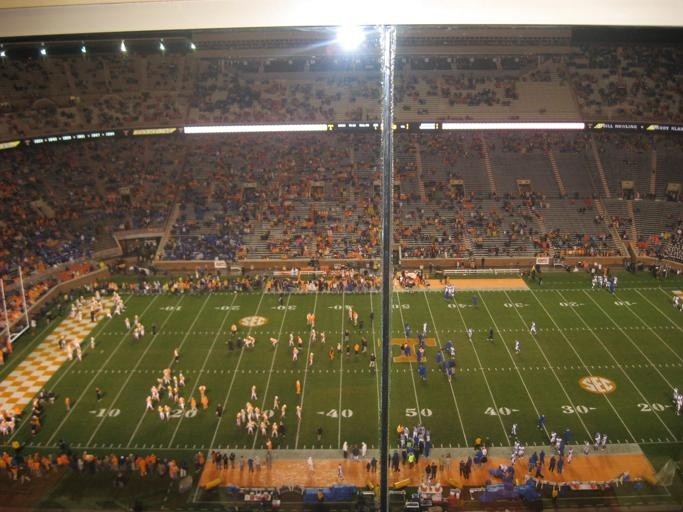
[1,39,683,511]
